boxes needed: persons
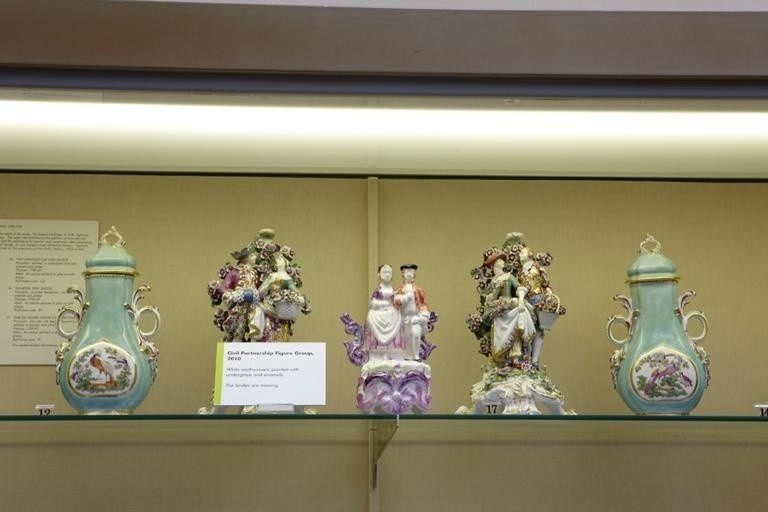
[364,264,404,360]
[484,249,528,374]
[514,248,552,368]
[256,254,306,342]
[214,248,264,341]
[393,264,430,362]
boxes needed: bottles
[609,236,712,419]
[51,223,162,416]
